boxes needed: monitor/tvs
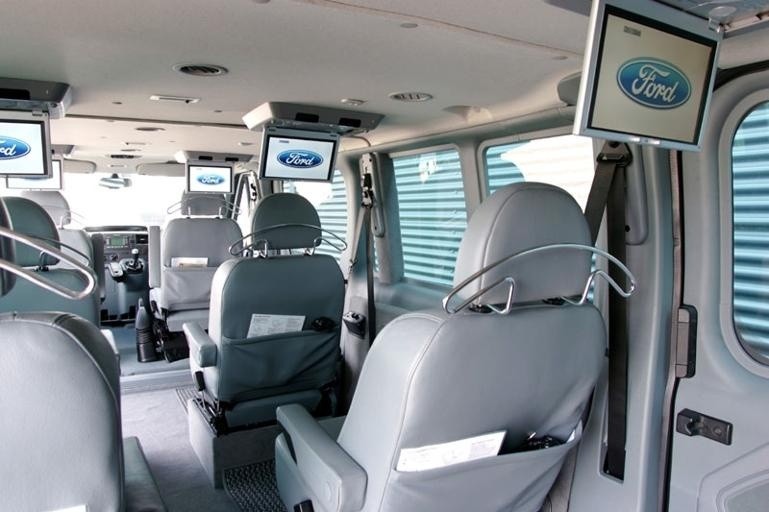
[572,0,722,153]
[0,109,53,179]
[6,155,62,190]
[257,126,340,182]
[185,161,234,194]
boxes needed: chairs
[271,183,606,511]
[21,190,106,308]
[180,191,348,495]
[0,195,122,379]
[0,195,171,511]
[145,189,245,362]
